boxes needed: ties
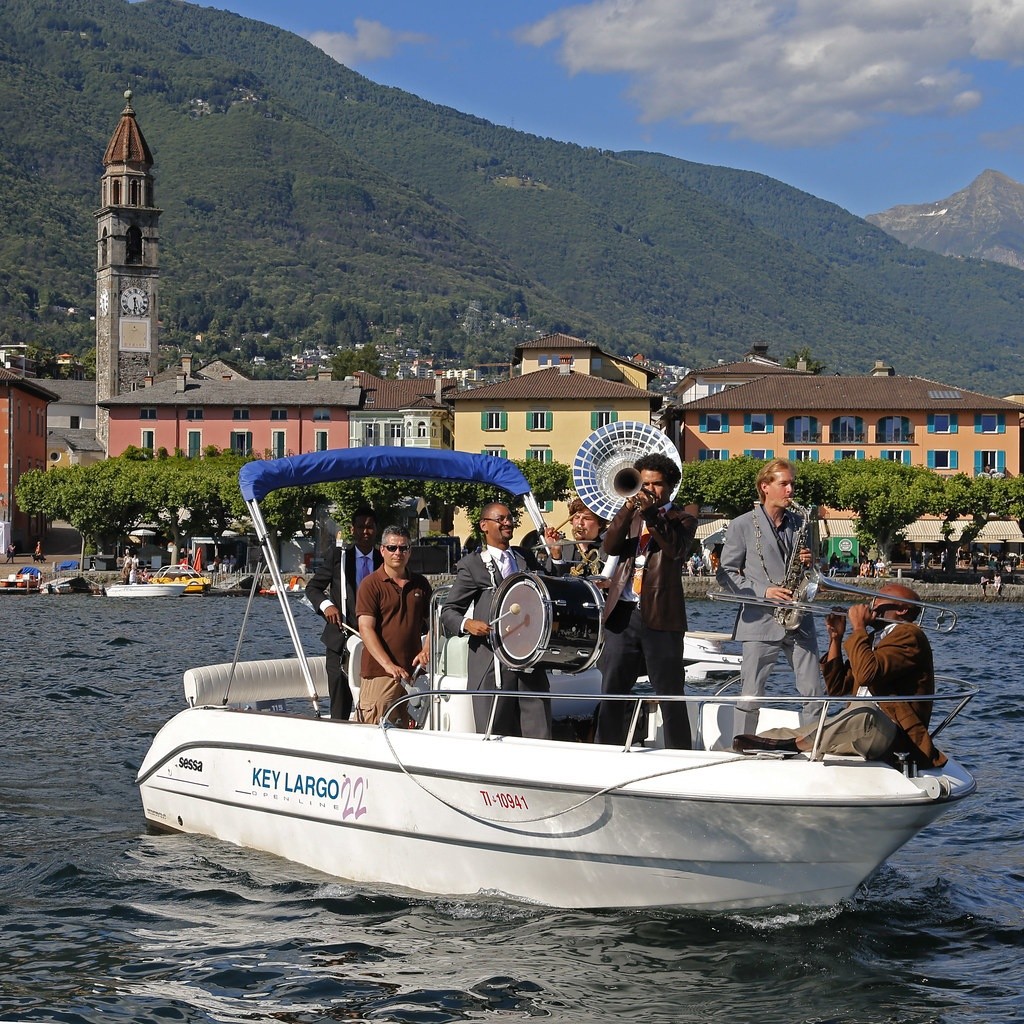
[503,551,514,578]
[359,556,370,578]
[632,526,650,595]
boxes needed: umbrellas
[193,546,202,573]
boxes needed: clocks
[99,288,108,318]
[121,286,149,317]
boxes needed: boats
[136,446,975,925]
[0,565,310,598]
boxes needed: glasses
[482,514,513,525]
[382,545,410,552]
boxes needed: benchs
[184,655,329,720]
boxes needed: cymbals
[531,539,597,550]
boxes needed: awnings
[693,518,828,539]
[827,520,1024,542]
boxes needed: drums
[489,572,607,677]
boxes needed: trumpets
[613,469,660,512]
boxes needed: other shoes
[732,734,795,759]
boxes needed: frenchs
[570,420,684,577]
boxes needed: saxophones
[773,498,826,630]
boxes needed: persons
[687,551,720,576]
[5,543,15,564]
[860,551,885,578]
[980,572,1002,598]
[957,545,1012,572]
[911,548,940,574]
[441,498,606,742]
[733,584,948,768]
[121,548,150,584]
[213,555,237,573]
[355,526,436,728]
[829,552,849,577]
[35,541,47,563]
[721,458,822,751]
[593,453,699,749]
[304,508,385,721]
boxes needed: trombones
[704,567,958,638]
